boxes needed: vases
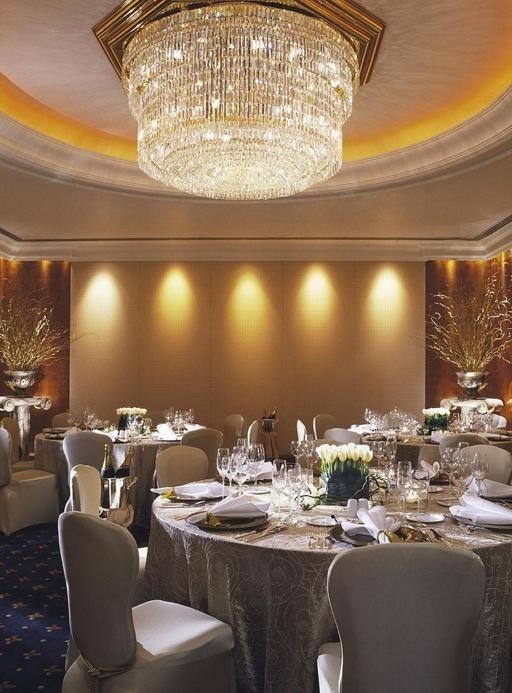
[455,370,489,400]
[2,368,41,397]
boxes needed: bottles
[116,446,137,476]
[101,442,115,477]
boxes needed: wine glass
[214,431,488,525]
[360,406,497,436]
[66,407,194,432]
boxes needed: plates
[166,481,511,546]
[41,427,192,442]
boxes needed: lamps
[92,0,388,202]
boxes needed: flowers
[314,442,374,474]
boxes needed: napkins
[453,492,509,528]
[344,504,398,546]
[188,492,263,526]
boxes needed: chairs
[32,405,254,499]
[0,427,59,534]
[316,542,485,692]
[70,463,154,598]
[293,405,511,478]
[58,511,235,692]
